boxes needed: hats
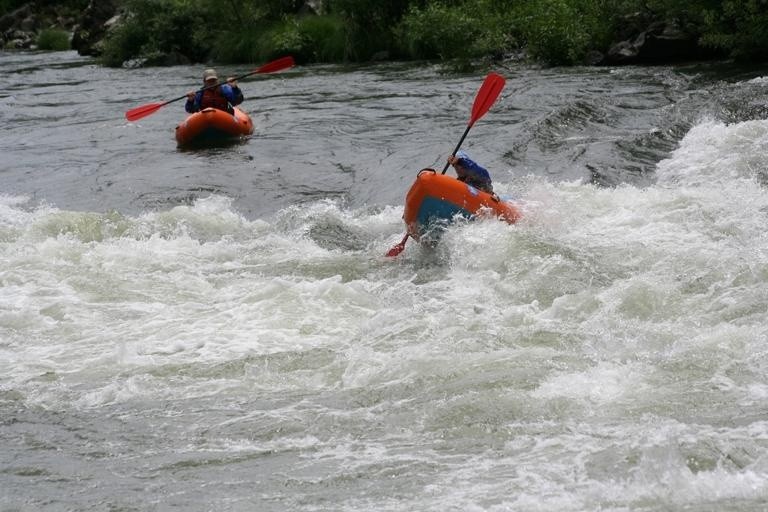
[203,69,218,81]
[449,151,469,167]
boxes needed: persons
[446,150,493,196]
[184,68,245,117]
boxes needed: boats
[174,105,254,144]
[399,165,528,253]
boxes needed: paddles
[126,57,293,121]
[386,72,505,256]
